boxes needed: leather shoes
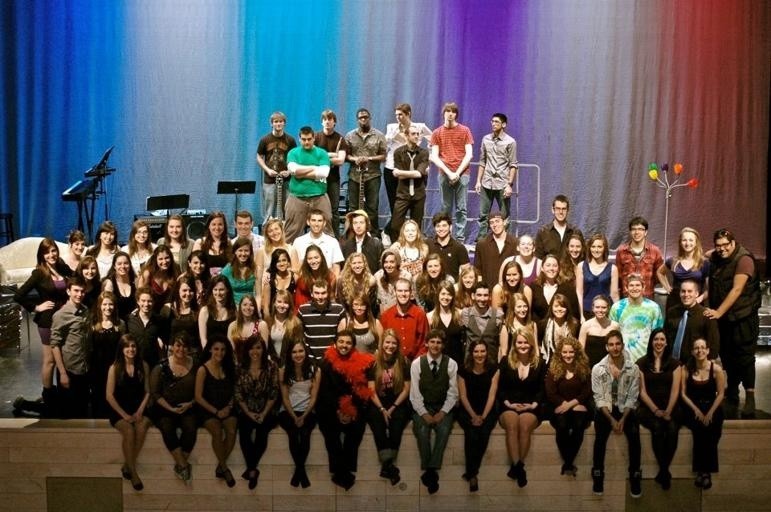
[122,465,130,479]
[694,470,712,490]
[131,474,144,490]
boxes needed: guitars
[339,164,373,249]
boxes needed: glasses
[715,242,732,248]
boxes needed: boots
[741,391,756,416]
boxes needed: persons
[283,126,335,245]
[536,195,583,259]
[257,111,297,227]
[388,127,429,245]
[429,102,474,245]
[314,110,349,241]
[14,208,764,500]
[385,102,433,215]
[344,107,388,240]
[475,114,519,243]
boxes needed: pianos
[61,179,97,200]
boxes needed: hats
[346,208,369,220]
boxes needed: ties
[671,310,689,361]
[406,151,417,196]
[431,361,437,374]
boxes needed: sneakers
[462,472,480,493]
[654,471,672,490]
[379,462,400,487]
[290,473,311,489]
[420,471,440,495]
[592,467,605,496]
[629,469,644,498]
[330,472,356,492]
[561,465,577,476]
[506,460,528,488]
[216,463,235,488]
[174,464,192,481]
[13,395,24,409]
[241,469,259,490]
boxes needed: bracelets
[509,181,515,187]
[369,157,373,160]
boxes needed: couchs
[0,237,88,289]
